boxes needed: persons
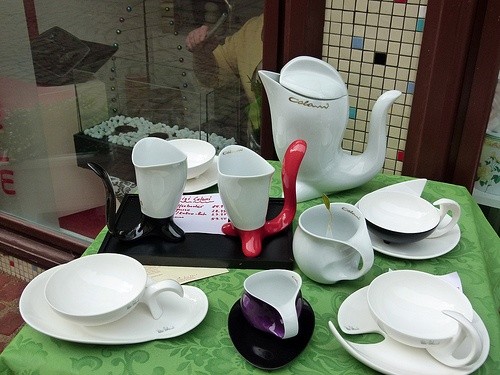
[186,12,263,152]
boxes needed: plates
[228,298,315,369]
[328,272,490,375]
[183,156,218,192]
[19,264,208,344]
[368,205,461,260]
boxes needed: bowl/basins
[166,138,216,178]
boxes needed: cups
[368,270,484,369]
[240,268,304,340]
[359,178,461,238]
[44,252,184,327]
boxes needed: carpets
[0,269,29,335]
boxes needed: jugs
[86,137,187,242]
[217,140,307,259]
[292,202,375,284]
[256,56,404,204]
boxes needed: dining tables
[0,159,500,375]
